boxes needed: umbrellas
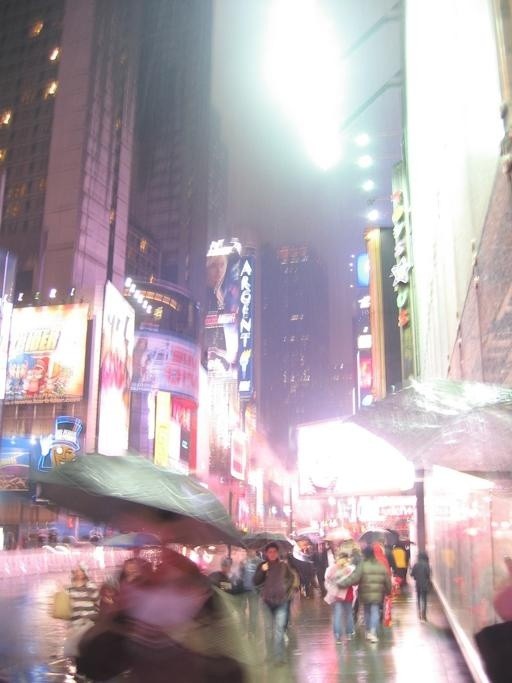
[342,375,512,486]
[25,453,244,547]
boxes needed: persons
[410,551,430,623]
[5,524,113,549]
[251,543,301,667]
[476,556,512,683]
[380,537,410,585]
[66,546,253,680]
[312,539,402,645]
[238,547,264,639]
[281,538,337,600]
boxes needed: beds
[25,453,244,547]
[342,375,512,486]
